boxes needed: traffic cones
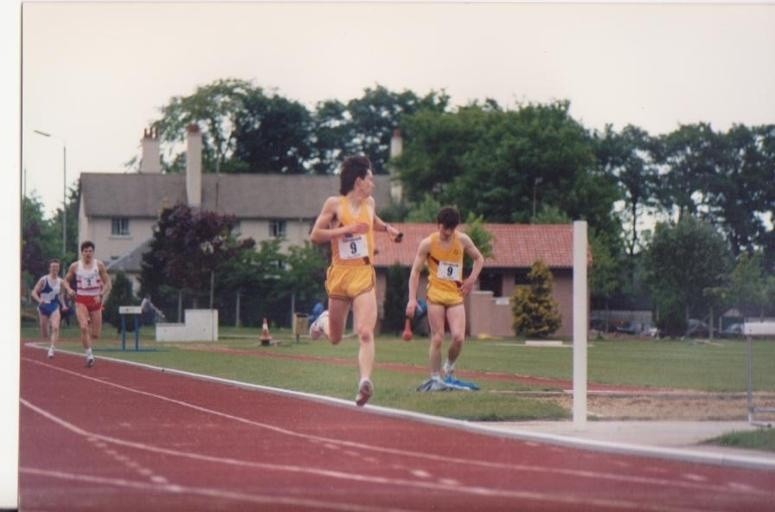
[259,317,272,341]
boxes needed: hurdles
[93,306,165,351]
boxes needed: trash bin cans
[294,312,309,335]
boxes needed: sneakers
[356,379,374,406]
[310,310,328,340]
[87,356,95,367]
[48,350,54,358]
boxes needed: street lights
[33,128,67,282]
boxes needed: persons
[406,208,484,376]
[31,260,67,359]
[63,241,111,367]
[309,156,404,405]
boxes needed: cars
[616,319,659,337]
[721,323,748,339]
[659,318,718,339]
